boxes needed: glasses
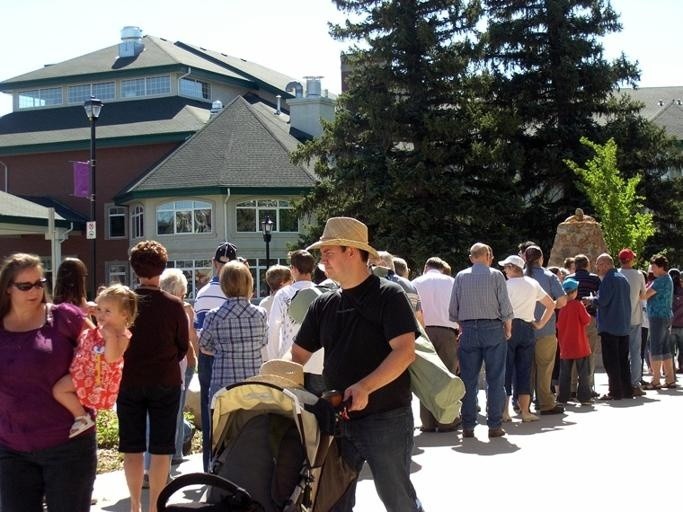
[7,278,48,291]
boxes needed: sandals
[68,412,95,439]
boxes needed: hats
[245,359,304,392]
[212,240,238,264]
[561,277,579,293]
[618,248,636,261]
[305,217,381,263]
[497,255,525,270]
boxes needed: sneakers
[141,426,203,488]
[420,368,683,438]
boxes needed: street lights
[79,92,105,300]
[257,212,277,295]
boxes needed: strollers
[155,361,351,512]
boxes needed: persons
[0,239,341,511]
[280,217,423,511]
[369,240,682,438]
